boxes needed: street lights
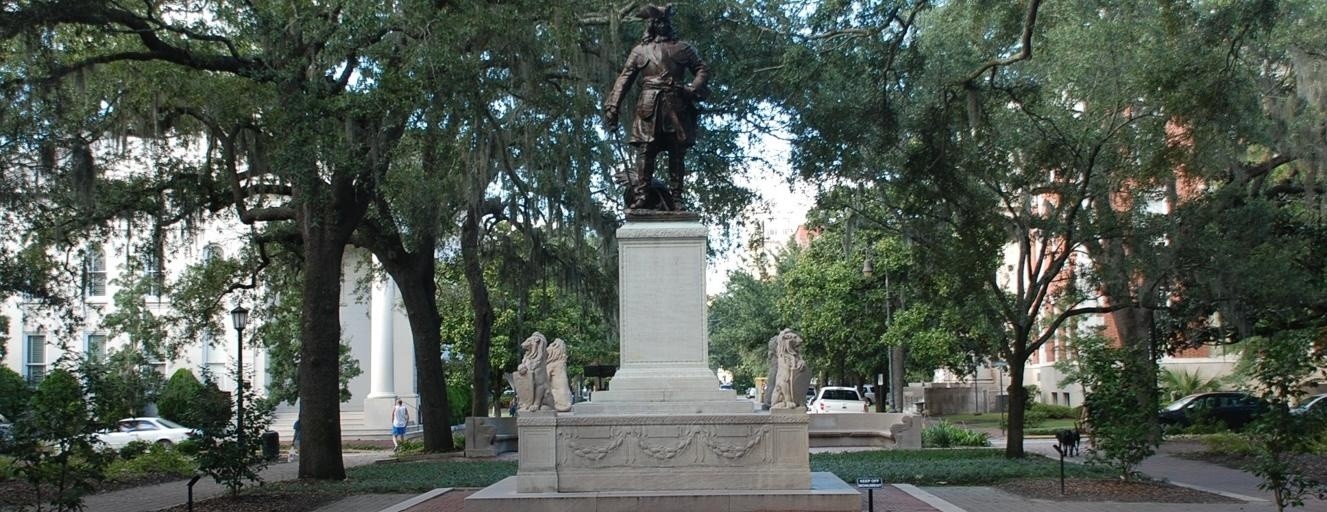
[231,302,249,443]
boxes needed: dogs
[1055,422,1080,457]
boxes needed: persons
[391,400,409,452]
[291,414,300,449]
[1077,382,1098,447]
[745,384,756,399]
[582,385,587,392]
[509,396,518,417]
[601,2,709,211]
[591,383,597,392]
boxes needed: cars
[811,386,869,415]
[1158,390,1271,443]
[88,415,204,459]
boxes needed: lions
[764,327,807,410]
[517,331,573,412]
